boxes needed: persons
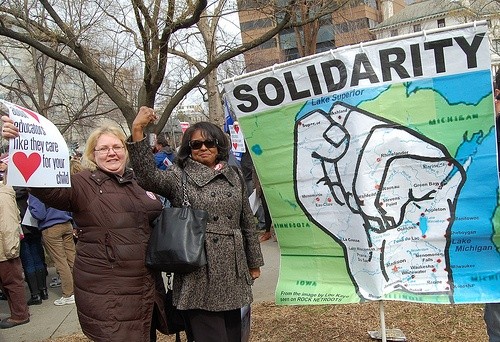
[484,68,500,342]
[1,114,170,342]
[0,145,83,329]
[152,135,176,170]
[233,121,278,244]
[125,106,264,342]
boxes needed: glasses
[189,138,218,150]
[94,146,124,154]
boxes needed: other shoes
[0,294,7,300]
[260,232,270,242]
[0,317,30,329]
[273,235,277,242]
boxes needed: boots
[24,271,43,305]
[37,268,49,300]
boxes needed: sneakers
[50,277,61,287]
[54,294,75,305]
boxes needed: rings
[3,128,5,132]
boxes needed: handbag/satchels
[164,289,185,334]
[144,208,208,274]
